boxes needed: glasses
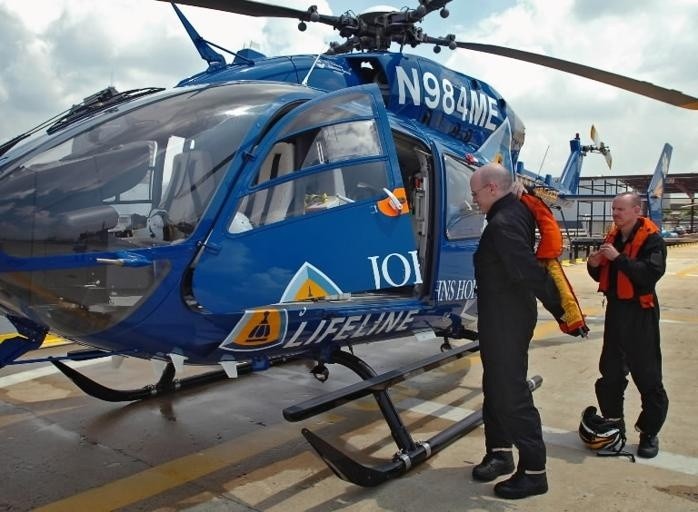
[471,184,488,196]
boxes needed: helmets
[578,407,620,450]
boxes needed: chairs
[156,141,297,230]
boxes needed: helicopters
[0,1,698,488]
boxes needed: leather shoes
[603,420,625,433]
[638,433,659,459]
[472,451,515,482]
[494,467,548,500]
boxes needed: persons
[586,193,670,460]
[470,162,592,500]
